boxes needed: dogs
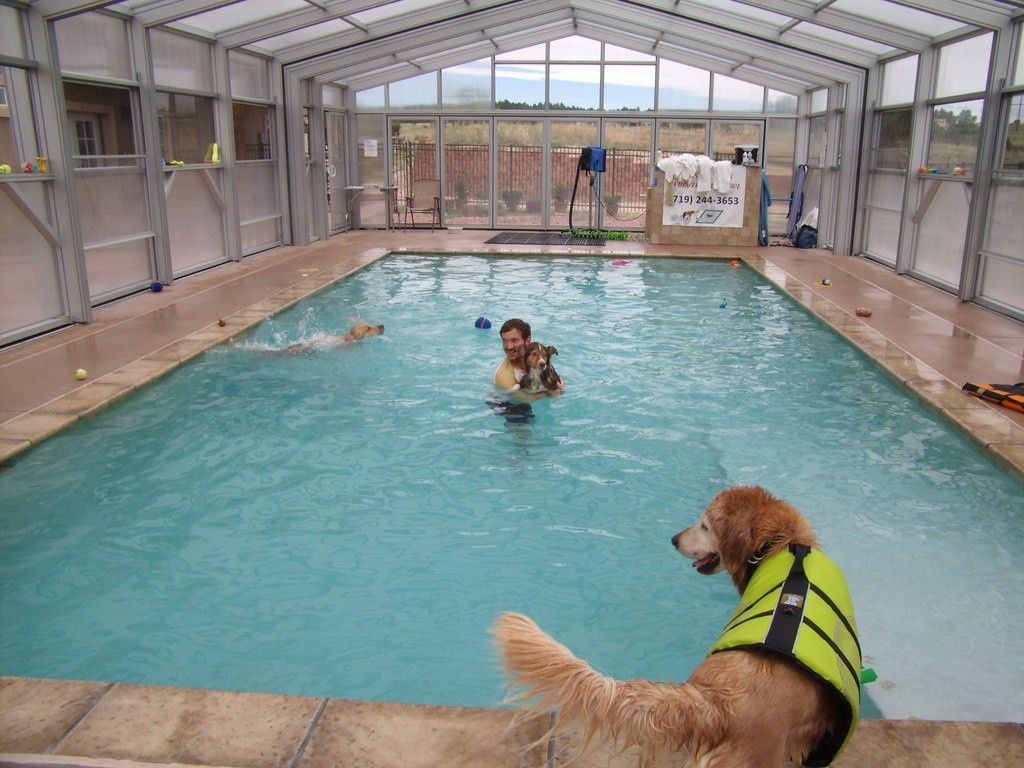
[510,342,561,393]
[283,323,385,353]
[487,486,837,768]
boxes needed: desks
[344,185,400,233]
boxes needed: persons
[494,318,565,404]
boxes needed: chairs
[404,179,442,233]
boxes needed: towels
[713,160,732,193]
[657,153,717,193]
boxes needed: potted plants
[604,194,622,217]
[504,189,523,212]
[526,194,541,214]
[454,181,468,211]
[551,182,568,213]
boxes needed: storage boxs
[735,146,758,164]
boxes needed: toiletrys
[657,149,662,164]
[650,163,658,186]
